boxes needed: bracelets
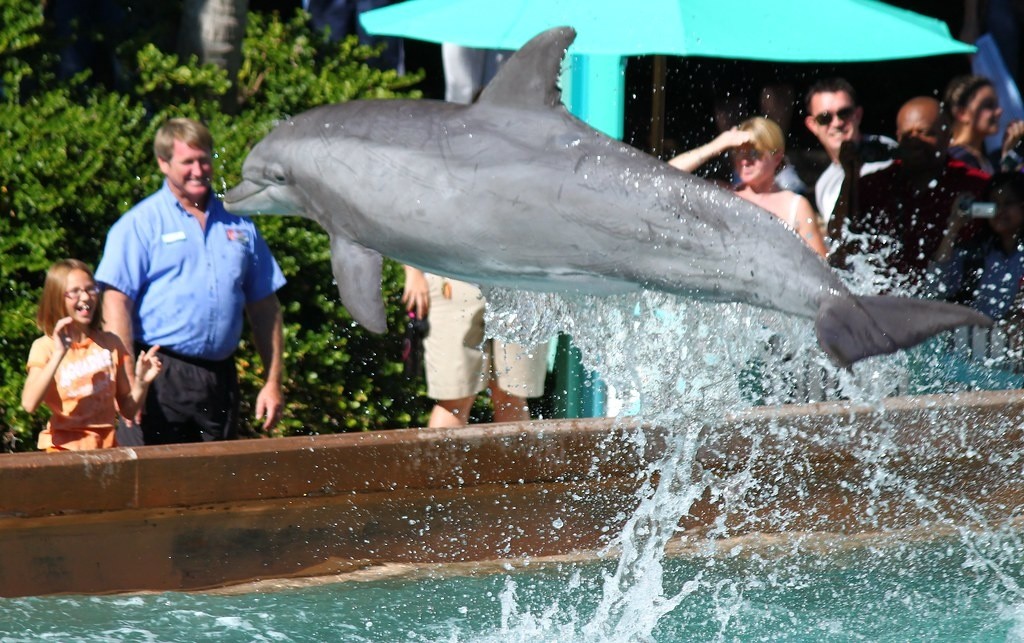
[689,150,701,166]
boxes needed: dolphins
[222,27,995,369]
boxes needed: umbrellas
[359,1,979,164]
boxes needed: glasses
[64,285,101,299]
[814,106,855,125]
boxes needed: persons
[21,259,162,451]
[94,118,288,445]
[665,51,1024,405]
[404,262,551,429]
